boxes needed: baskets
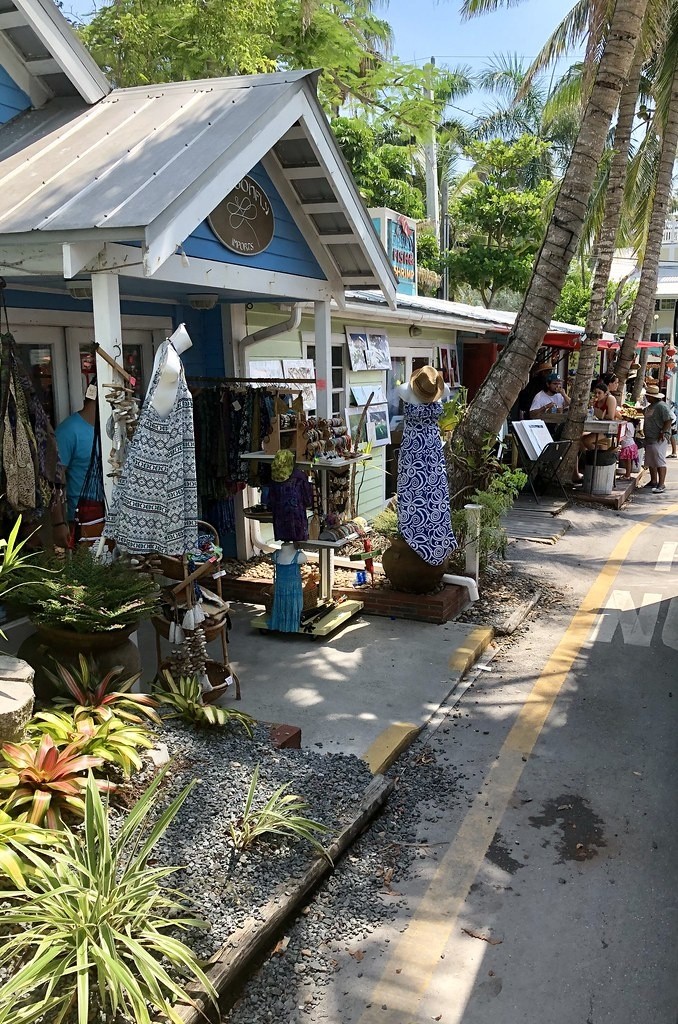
[157,658,234,704]
[157,520,222,581]
[260,584,318,615]
[152,617,226,646]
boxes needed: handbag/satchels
[66,496,106,549]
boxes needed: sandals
[619,475,630,480]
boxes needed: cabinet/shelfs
[508,418,573,504]
[237,448,372,638]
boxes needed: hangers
[186,372,300,399]
[88,337,138,386]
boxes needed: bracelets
[543,405,547,409]
[52,522,66,528]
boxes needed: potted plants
[373,500,468,592]
[12,542,161,701]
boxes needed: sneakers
[654,485,666,492]
[644,481,658,488]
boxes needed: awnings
[496,329,664,350]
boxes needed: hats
[644,385,665,399]
[619,408,638,419]
[535,363,556,375]
[410,365,445,403]
[270,450,295,483]
[546,374,565,382]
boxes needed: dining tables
[616,412,645,470]
[525,412,627,498]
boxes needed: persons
[49,375,106,548]
[642,385,678,492]
[266,543,307,632]
[522,363,571,419]
[396,365,458,566]
[266,449,312,544]
[100,324,198,555]
[581,372,639,480]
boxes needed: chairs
[592,412,621,459]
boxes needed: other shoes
[666,455,677,458]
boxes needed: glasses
[609,373,617,380]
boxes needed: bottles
[550,398,558,414]
[588,406,595,422]
